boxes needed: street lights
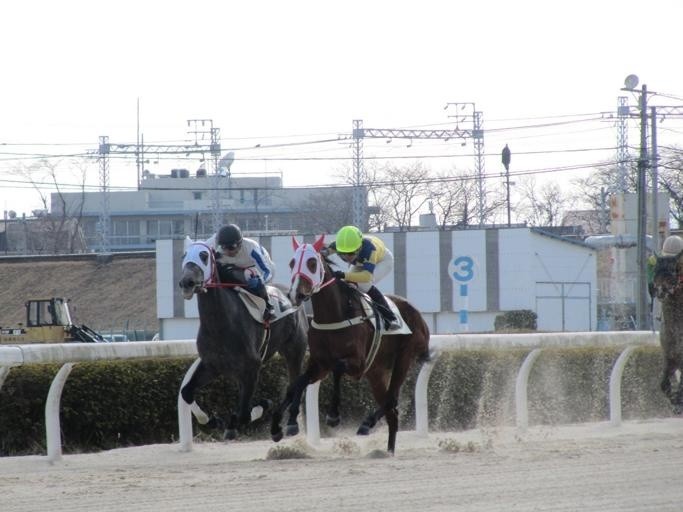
[501,142,511,224]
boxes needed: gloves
[248,278,261,288]
[334,271,344,279]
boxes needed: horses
[269,233,430,456]
[646,249,682,414]
[177,233,310,444]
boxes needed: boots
[367,286,396,331]
[251,285,276,320]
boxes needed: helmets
[335,226,362,253]
[217,224,242,245]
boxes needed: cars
[100,333,129,343]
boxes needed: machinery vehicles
[0,297,112,344]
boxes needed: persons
[661,235,683,277]
[320,225,403,331]
[213,224,275,328]
